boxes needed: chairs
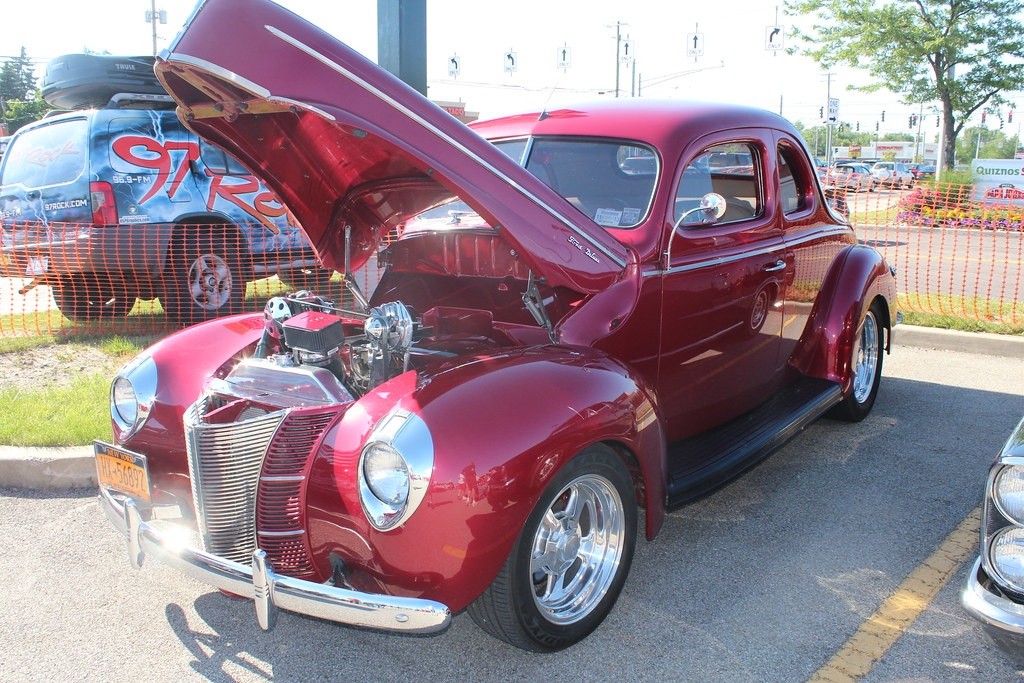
[582,198,629,210]
[671,200,752,226]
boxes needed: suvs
[1,51,335,328]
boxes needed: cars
[91,0,906,655]
[960,410,1024,660]
[621,156,970,193]
